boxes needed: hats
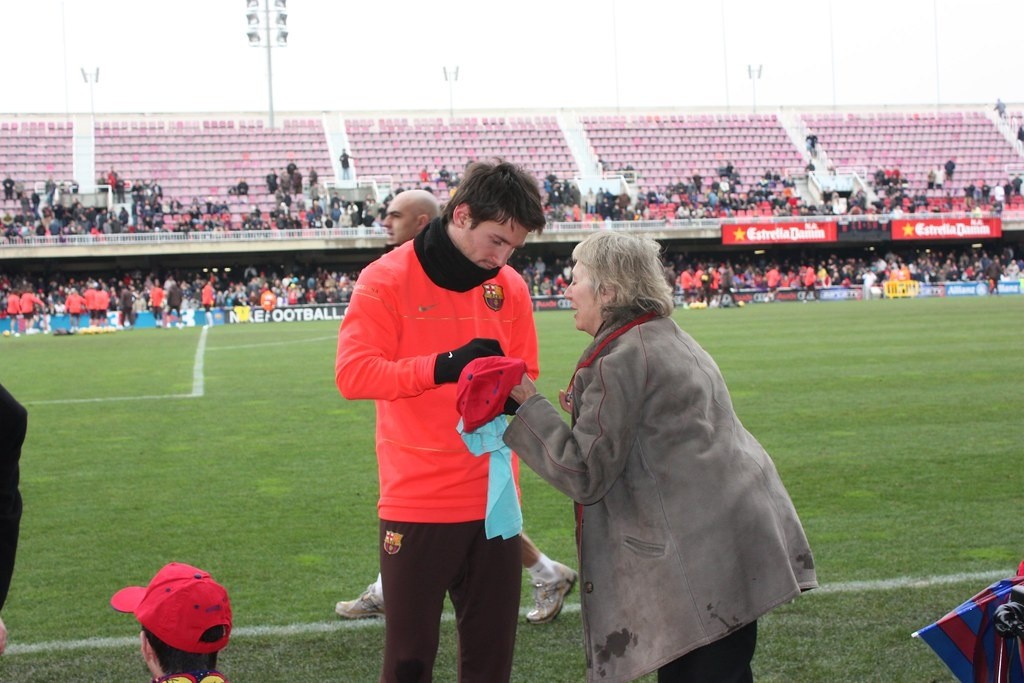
[454,357,527,433]
[110,562,233,653]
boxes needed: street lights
[246,0,287,128]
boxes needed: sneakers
[335,583,386,618]
[526,561,578,624]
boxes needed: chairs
[0,106,1023,246]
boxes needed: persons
[0,171,263,244]
[0,384,28,651]
[816,243,1024,296]
[674,161,812,227]
[506,250,576,309]
[335,159,547,683]
[991,97,1006,117]
[111,561,234,683]
[197,266,362,326]
[502,233,820,683]
[266,147,461,238]
[661,251,821,309]
[538,162,676,230]
[1016,124,1024,154]
[336,190,577,625]
[0,270,201,337]
[805,133,1023,218]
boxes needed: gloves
[501,396,520,415]
[435,338,505,384]
